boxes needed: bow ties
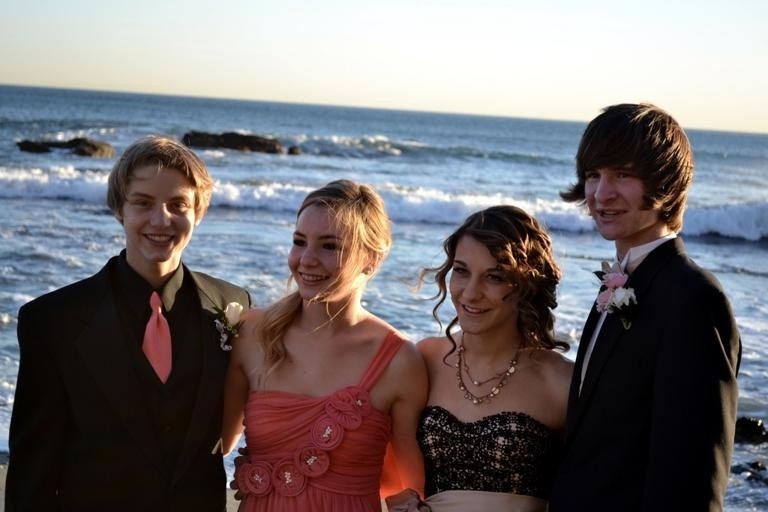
[600,261,625,277]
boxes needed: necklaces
[457,330,527,406]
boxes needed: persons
[403,204,576,511]
[0,132,258,510]
[220,174,432,509]
[551,99,744,509]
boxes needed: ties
[139,291,173,387]
